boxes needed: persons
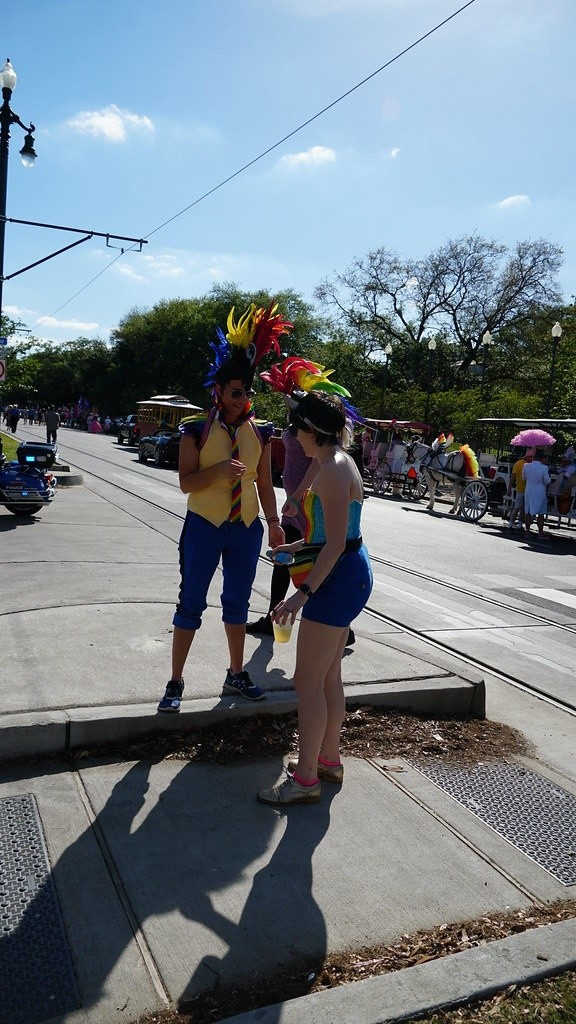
[246,390,355,647]
[158,353,285,713]
[505,446,576,540]
[0,404,112,445]
[257,389,373,805]
[160,410,173,427]
[362,426,373,447]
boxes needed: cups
[270,611,292,642]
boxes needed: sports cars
[139,429,182,465]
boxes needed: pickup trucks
[118,414,155,446]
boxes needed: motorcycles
[0,437,60,516]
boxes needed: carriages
[348,418,576,527]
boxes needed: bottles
[266,550,294,564]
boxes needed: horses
[410,444,480,513]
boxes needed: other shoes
[525,532,533,537]
[506,527,512,534]
[538,533,550,540]
[246,615,276,635]
[345,630,354,645]
[516,526,525,533]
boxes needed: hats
[522,450,533,458]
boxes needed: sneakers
[158,675,185,712]
[289,756,344,785]
[224,667,264,700]
[256,765,321,805]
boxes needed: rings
[285,607,291,613]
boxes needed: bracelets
[268,517,279,525]
[287,496,300,509]
[300,583,314,598]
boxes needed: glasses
[224,389,257,402]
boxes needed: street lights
[381,342,393,417]
[546,321,562,417]
[424,338,437,422]
[480,330,493,394]
[0,56,38,348]
